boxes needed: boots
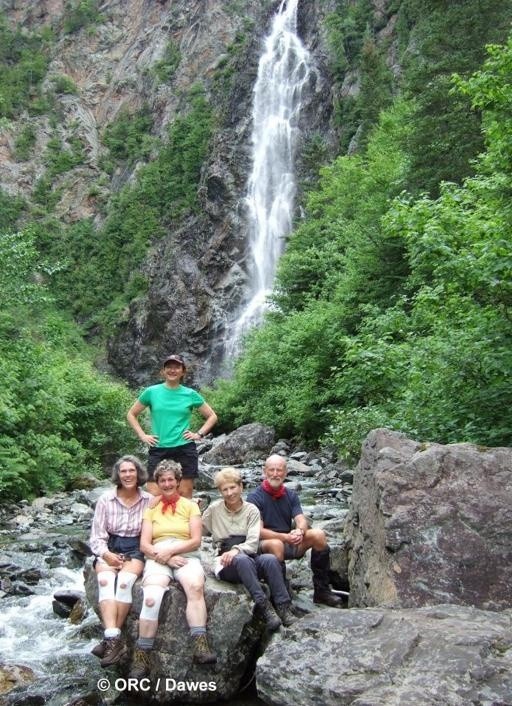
[309,546,344,606]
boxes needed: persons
[246,454,343,613]
[147,466,299,630]
[128,458,218,679]
[89,454,156,668]
[126,354,218,499]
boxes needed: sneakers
[127,645,153,682]
[189,633,219,665]
[256,598,282,633]
[90,634,129,668]
[275,599,301,627]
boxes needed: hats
[163,353,187,368]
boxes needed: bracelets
[299,528,305,537]
[197,431,203,438]
